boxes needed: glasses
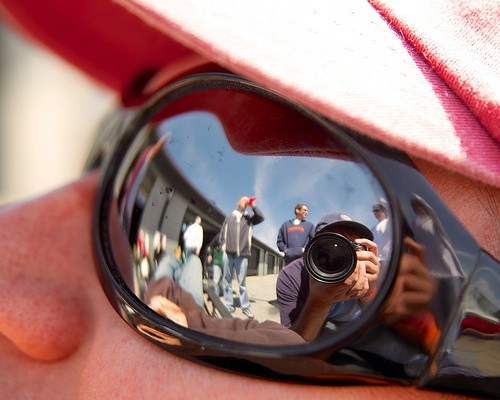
[372,209,380,213]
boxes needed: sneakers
[243,308,254,318]
[226,306,235,313]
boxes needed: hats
[314,212,374,242]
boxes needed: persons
[0,0,500,400]
[118,195,471,378]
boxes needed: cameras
[302,233,369,284]
